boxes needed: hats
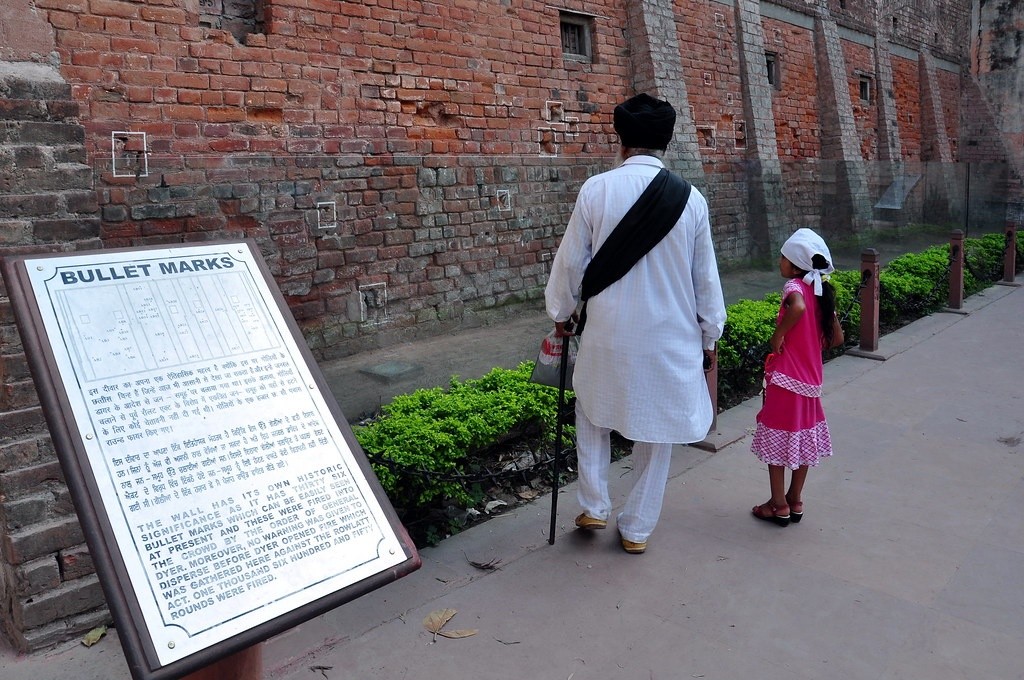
[613,93,677,150]
[780,227,835,296]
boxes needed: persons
[547,92,729,557]
[746,224,843,530]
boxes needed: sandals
[785,502,804,523]
[751,499,790,527]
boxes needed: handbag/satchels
[528,320,580,391]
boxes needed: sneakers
[574,513,607,529]
[616,515,647,553]
